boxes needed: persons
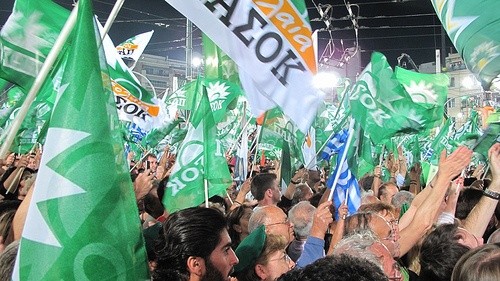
[0,140,500,280]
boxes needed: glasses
[387,217,399,226]
[264,218,290,228]
[262,253,290,266]
[378,227,400,243]
[21,175,33,180]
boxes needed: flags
[1,0,500,222]
[12,1,152,281]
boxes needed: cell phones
[145,160,150,176]
